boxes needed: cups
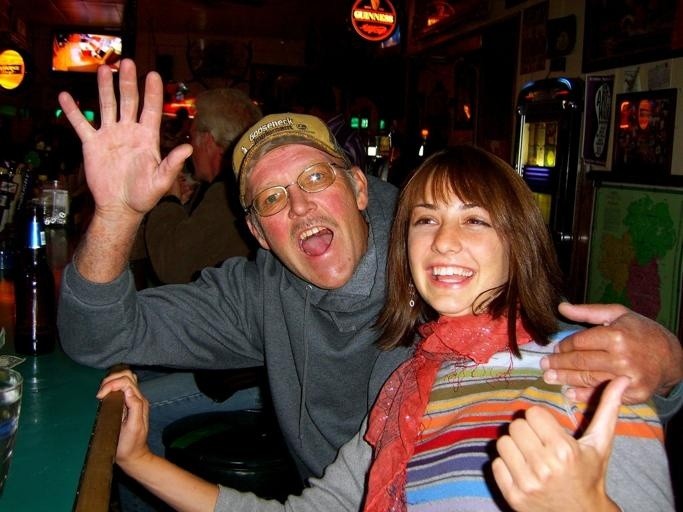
[0,367,23,500]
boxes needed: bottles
[0,162,53,358]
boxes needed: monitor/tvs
[51,32,123,74]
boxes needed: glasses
[243,161,345,218]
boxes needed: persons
[97,145,675,512]
[137,88,264,461]
[56,57,683,495]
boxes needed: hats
[231,112,349,207]
